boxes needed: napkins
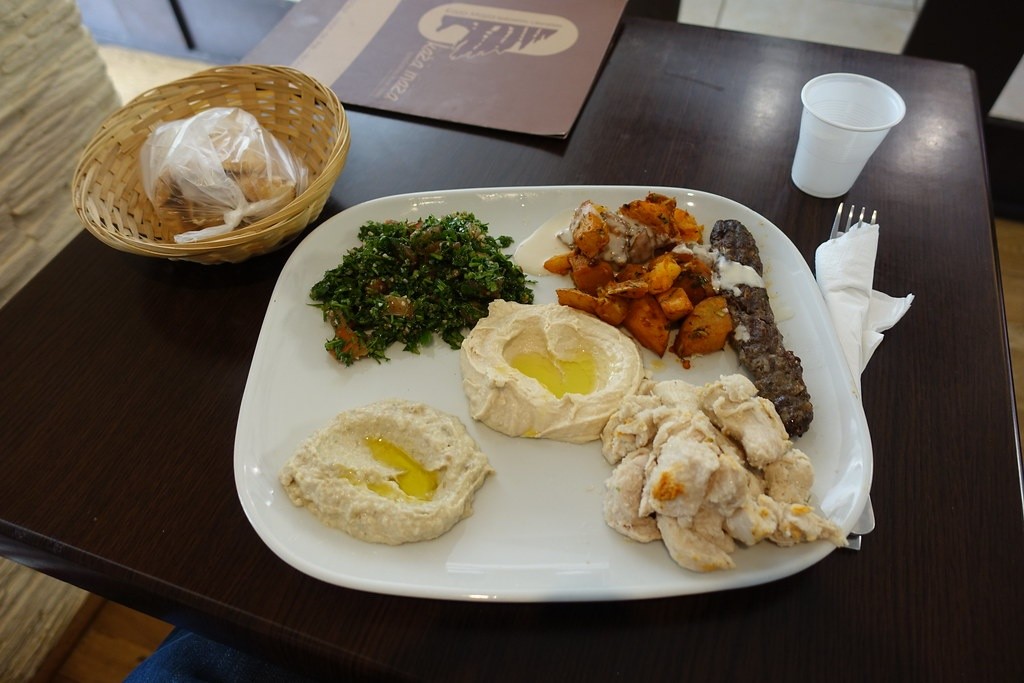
[813,223,918,537]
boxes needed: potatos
[543,192,732,358]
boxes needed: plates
[234,184,874,603]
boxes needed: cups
[791,72,906,199]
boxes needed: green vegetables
[308,210,538,367]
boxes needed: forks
[827,201,877,241]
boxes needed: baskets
[71,63,352,265]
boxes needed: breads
[154,132,297,228]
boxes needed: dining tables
[1,12,1024,683]
